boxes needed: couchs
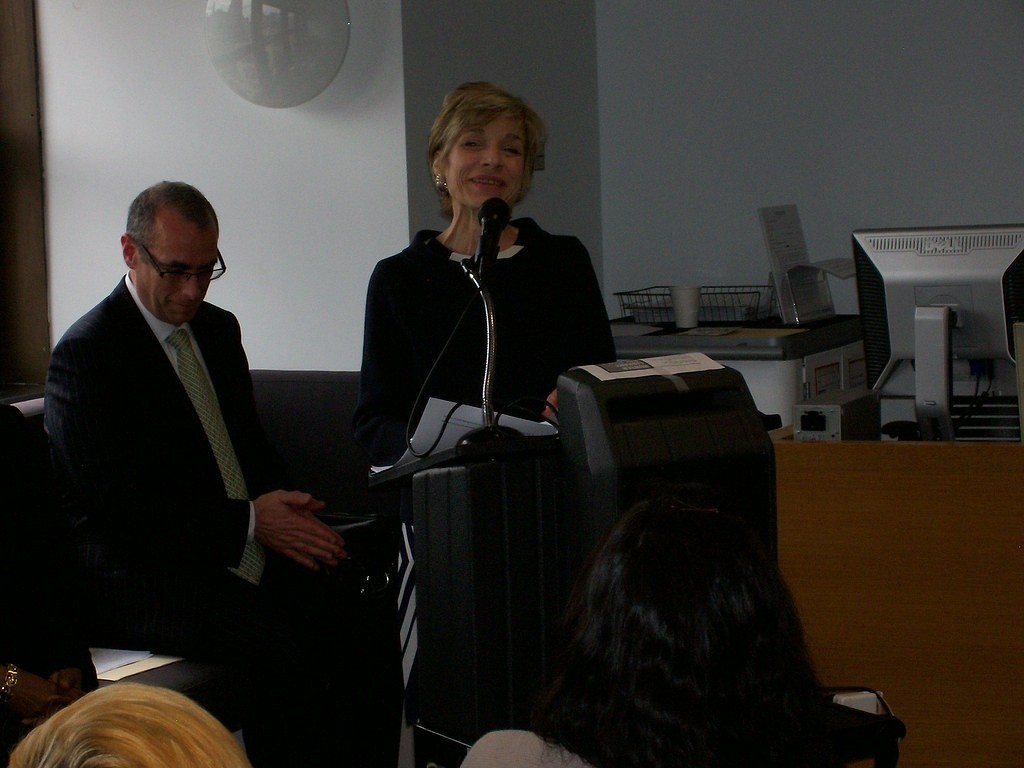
[10,369,362,690]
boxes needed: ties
[165,328,267,585]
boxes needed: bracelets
[0,663,19,703]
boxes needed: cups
[669,286,702,329]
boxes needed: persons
[0,402,99,768]
[350,84,616,767]
[9,681,252,766]
[43,179,403,767]
[461,483,832,767]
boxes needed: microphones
[477,197,510,286]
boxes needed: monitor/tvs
[851,222,1024,443]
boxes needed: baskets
[614,286,776,327]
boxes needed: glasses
[140,244,226,283]
